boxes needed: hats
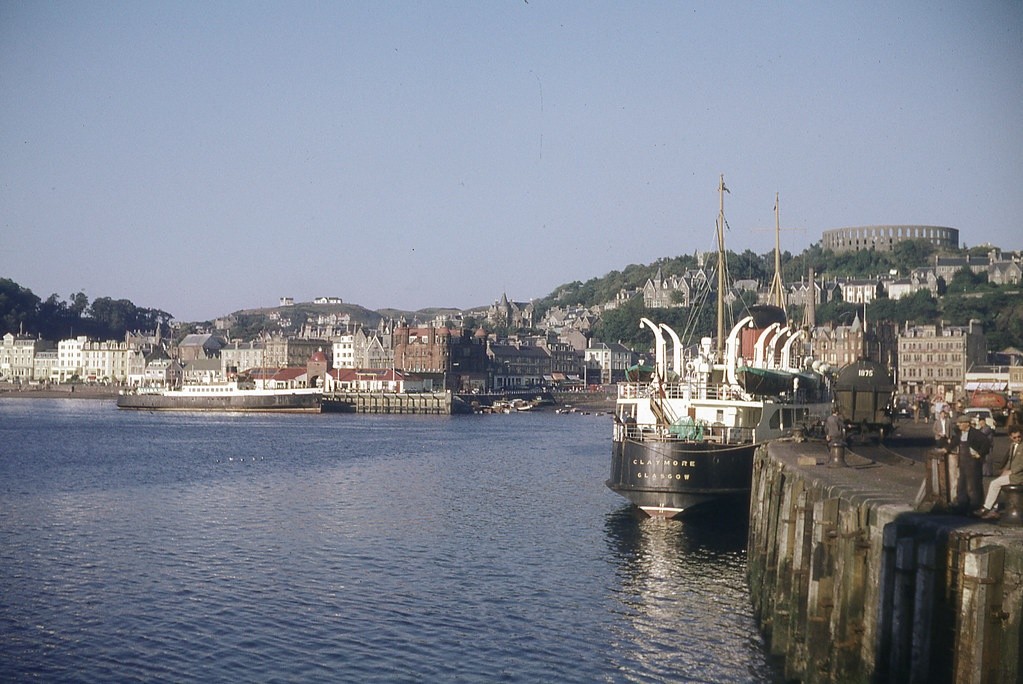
[955,415,971,423]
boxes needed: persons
[976,418,993,477]
[823,406,851,453]
[625,412,637,440]
[932,405,953,448]
[973,424,1023,521]
[911,393,931,424]
[934,415,989,516]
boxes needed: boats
[116,324,323,414]
[606,174,835,521]
[478,397,613,418]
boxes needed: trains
[832,357,894,446]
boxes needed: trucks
[973,390,1008,424]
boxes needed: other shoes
[956,505,969,512]
[981,509,1000,519]
[973,506,986,515]
[970,505,981,514]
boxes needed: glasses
[1012,435,1022,440]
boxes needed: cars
[898,403,910,417]
[962,408,996,433]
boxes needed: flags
[659,375,666,398]
[773,196,776,210]
[722,177,730,193]
